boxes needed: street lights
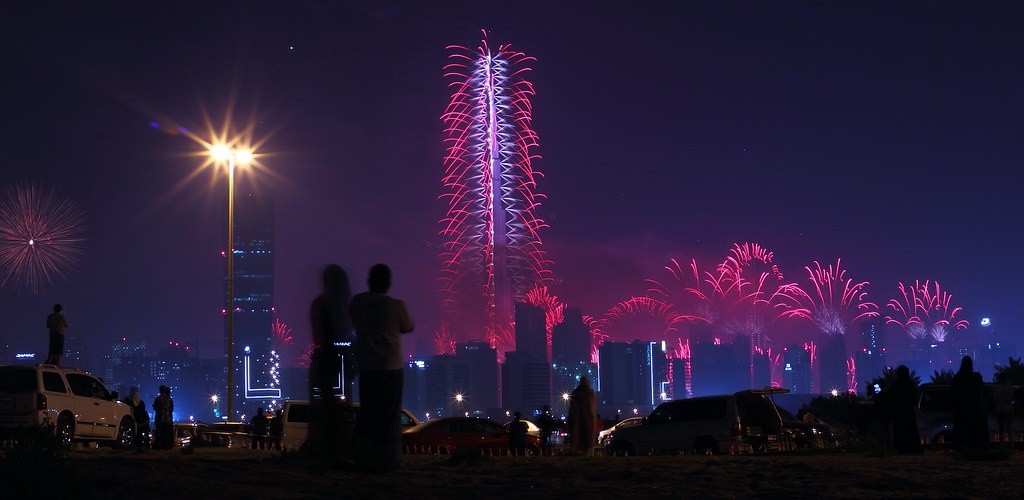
[205,135,252,424]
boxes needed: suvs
[0,363,138,451]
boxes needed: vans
[914,382,1024,449]
[279,400,420,452]
[609,388,791,459]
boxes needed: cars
[785,420,874,454]
[598,417,645,447]
[401,416,575,460]
[172,422,204,447]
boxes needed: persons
[536,405,552,457]
[296,265,355,470]
[948,356,993,449]
[508,411,527,456]
[123,385,175,450]
[889,365,922,456]
[250,409,284,451]
[994,379,1024,450]
[567,377,604,461]
[44,304,68,369]
[349,264,414,472]
